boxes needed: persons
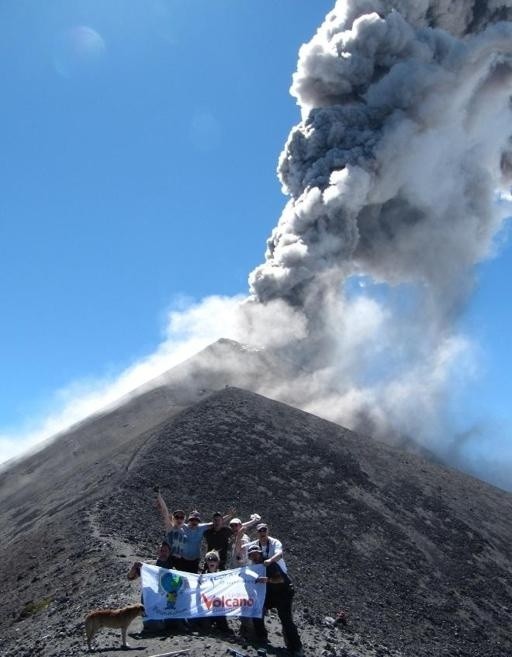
[128,489,302,656]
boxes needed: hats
[229,517,241,525]
[211,511,223,518]
[256,523,268,532]
[204,549,221,562]
[173,510,186,515]
[247,545,263,556]
[187,511,201,522]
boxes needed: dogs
[84,603,148,650]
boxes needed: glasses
[206,557,218,563]
[174,515,184,520]
